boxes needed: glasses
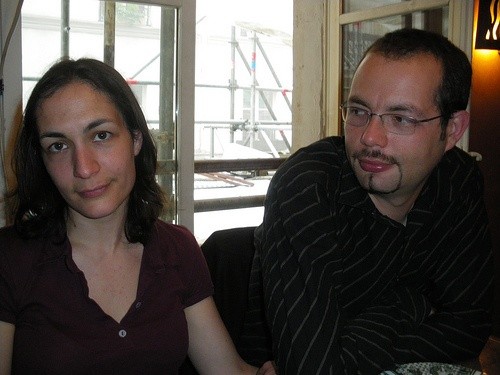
[338,101,449,136]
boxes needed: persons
[254,27,500,375]
[0,59,254,375]
